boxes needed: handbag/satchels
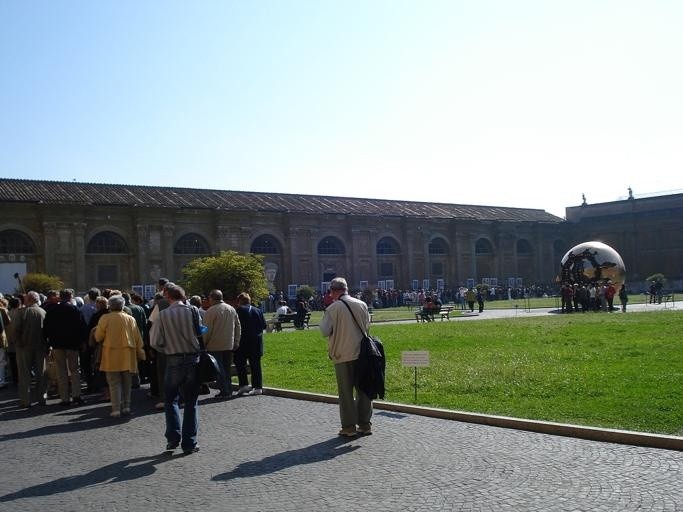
[191,306,221,384]
[75,310,89,341]
[361,335,382,373]
[46,350,57,381]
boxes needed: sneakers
[155,402,165,409]
[357,427,372,435]
[338,429,357,437]
[110,409,121,418]
[122,408,130,415]
[215,384,263,399]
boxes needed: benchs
[272,314,310,332]
[415,306,452,322]
[367,307,374,323]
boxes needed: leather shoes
[166,440,180,450]
[59,402,70,407]
[183,445,200,455]
[73,397,84,404]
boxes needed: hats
[330,276,348,290]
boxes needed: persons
[316,275,377,440]
[270,280,559,331]
[560,280,629,313]
[147,284,206,457]
[648,280,657,304]
[655,278,664,304]
[0,277,268,419]
